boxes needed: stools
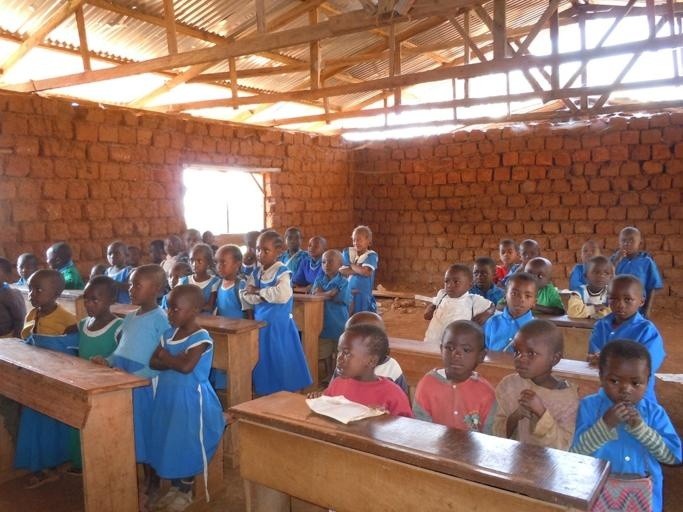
[316,336,340,383]
[159,411,235,503]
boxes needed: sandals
[156,487,180,510]
[166,490,194,511]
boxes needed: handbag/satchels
[591,473,653,511]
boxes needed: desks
[7,285,88,322]
[0,335,151,512]
[293,286,361,374]
[229,390,610,512]
[245,293,328,390]
[109,283,267,469]
[486,310,601,363]
[386,335,683,512]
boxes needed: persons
[13,269,77,492]
[146,284,222,510]
[240,229,313,396]
[307,324,413,418]
[279,225,379,312]
[567,338,683,512]
[424,227,665,356]
[0,229,259,383]
[329,312,408,392]
[91,263,165,512]
[413,322,496,434]
[583,273,665,402]
[65,276,124,474]
[495,319,579,448]
[311,248,348,387]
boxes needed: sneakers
[144,486,162,511]
[139,479,163,497]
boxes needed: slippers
[22,469,62,490]
[63,465,83,481]
